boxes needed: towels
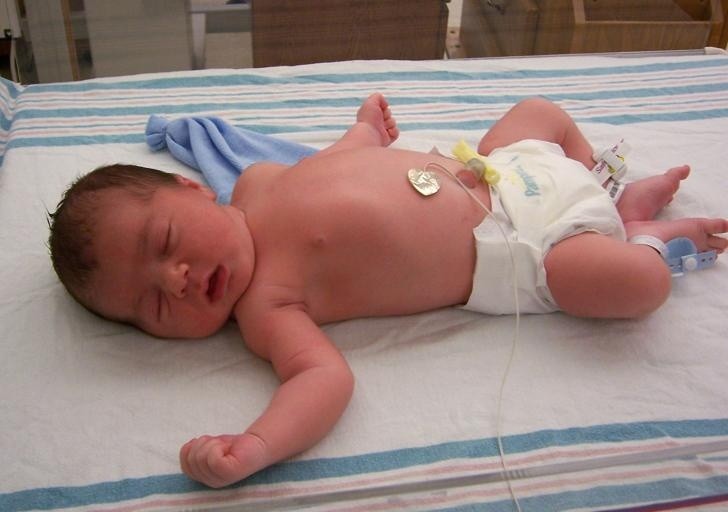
[144,108,320,205]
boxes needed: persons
[45,93,728,489]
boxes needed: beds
[0,46,727,512]
